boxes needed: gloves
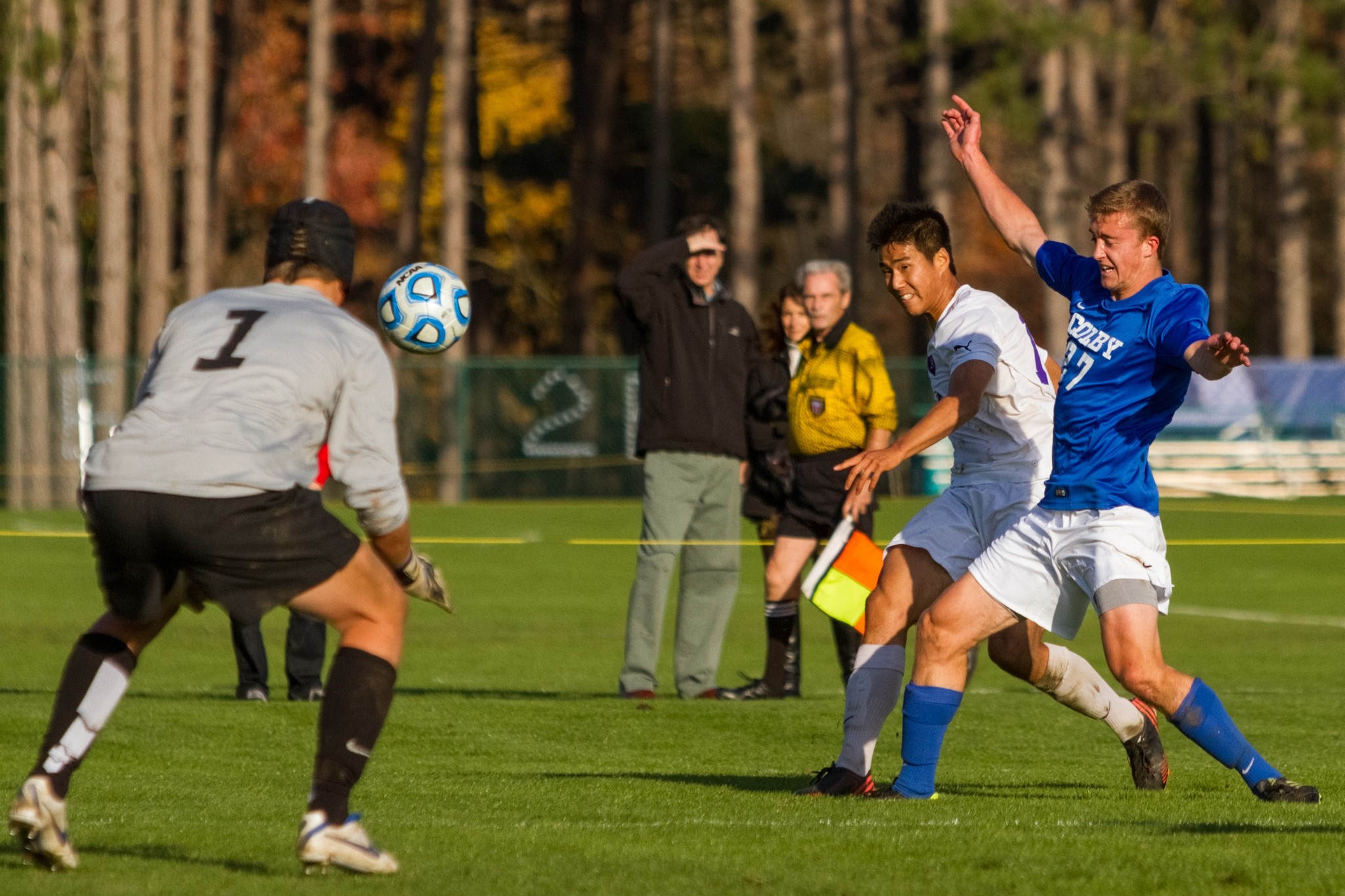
[392,547,454,611]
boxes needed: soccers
[376,261,472,356]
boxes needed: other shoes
[287,681,323,701]
[628,689,654,698]
[237,685,266,702]
[701,689,717,699]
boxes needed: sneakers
[1119,697,1169,791]
[866,777,938,800]
[1255,775,1321,804]
[295,809,398,874]
[792,759,876,797]
[8,775,78,873]
[714,670,799,700]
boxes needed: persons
[793,197,1169,790]
[7,196,450,878]
[868,91,1324,803]
[227,439,332,701]
[615,216,901,701]
